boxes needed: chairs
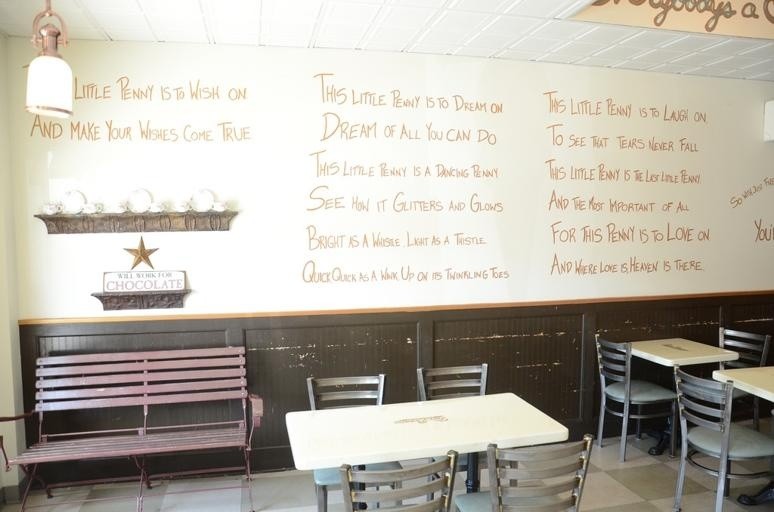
[308,373,403,511]
[668,366,774,512]
[341,447,459,512]
[705,326,772,432]
[487,433,593,511]
[416,362,488,501]
[591,334,678,461]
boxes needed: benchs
[0,347,264,512]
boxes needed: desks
[616,337,739,458]
[711,366,774,435]
[284,391,568,512]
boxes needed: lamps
[26,0,74,115]
